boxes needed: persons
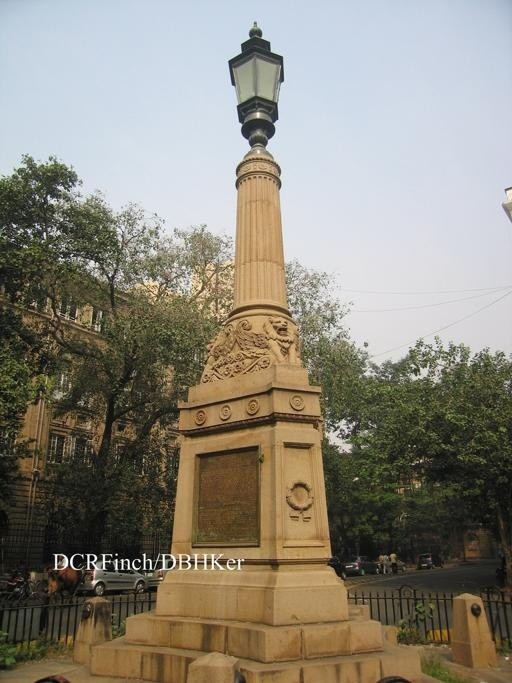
[15,573,24,586]
[48,577,58,604]
[58,553,66,568]
[496,544,502,560]
[379,551,398,575]
[438,552,448,567]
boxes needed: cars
[414,552,445,569]
[330,554,347,579]
[374,554,408,573]
[83,560,149,594]
[345,552,382,577]
[143,554,177,590]
